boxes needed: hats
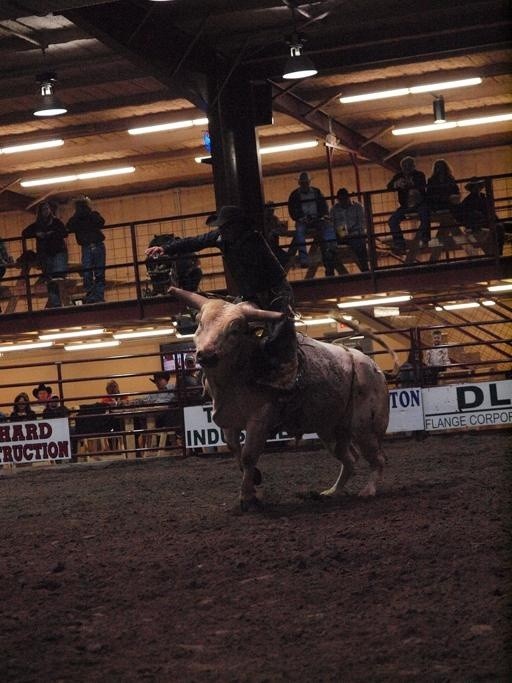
[33,383,52,399]
[295,173,312,182]
[465,177,486,190]
[184,355,195,363]
[149,373,170,383]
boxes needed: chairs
[72,403,177,462]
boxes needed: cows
[166,285,400,516]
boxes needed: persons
[355,330,449,388]
[149,356,212,456]
[66,197,107,305]
[100,378,129,456]
[144,203,299,407]
[10,384,74,467]
[330,187,369,274]
[262,199,293,279]
[21,201,69,308]
[386,156,505,259]
[288,172,340,267]
[146,232,204,296]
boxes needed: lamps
[278,0,321,80]
[432,96,446,124]
[29,38,68,118]
